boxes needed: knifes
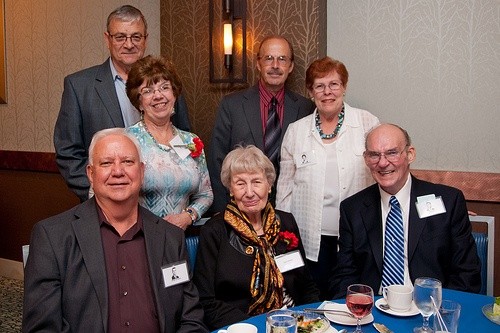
[372,323,393,333]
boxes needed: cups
[265,315,297,333]
[220,323,257,333]
[383,285,413,311]
[432,299,461,333]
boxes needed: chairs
[469,216,495,296]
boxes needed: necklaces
[315,104,345,139]
[142,118,176,151]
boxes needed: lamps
[222,23,234,69]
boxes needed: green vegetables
[291,314,322,333]
[486,297,500,317]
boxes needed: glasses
[107,31,146,42]
[366,144,408,163]
[312,81,343,93]
[138,84,173,97]
[258,55,291,64]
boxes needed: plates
[481,304,500,325]
[288,311,330,333]
[324,303,374,325]
[375,298,420,317]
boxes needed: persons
[301,155,309,164]
[22,6,483,333]
[171,267,178,279]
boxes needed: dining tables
[210,287,500,333]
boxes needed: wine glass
[346,284,374,333]
[413,277,443,333]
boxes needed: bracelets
[184,208,196,225]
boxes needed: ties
[381,195,405,296]
[264,96,282,207]
